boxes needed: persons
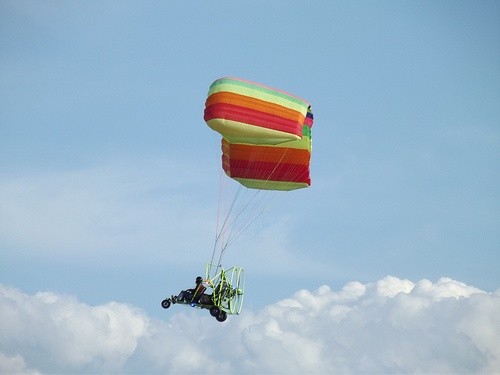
[179,276,203,303]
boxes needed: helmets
[195,277,202,285]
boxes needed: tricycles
[161,261,246,322]
